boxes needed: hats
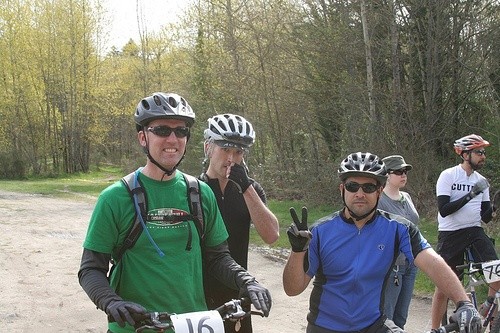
[382,154,412,172]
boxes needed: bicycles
[429,245,500,333]
[108,296,265,333]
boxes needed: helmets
[203,114,256,153]
[133,92,196,134]
[453,134,490,156]
[337,151,387,189]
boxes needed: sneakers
[429,327,445,333]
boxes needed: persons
[197,113,280,333]
[377,154,418,329]
[78,92,272,333]
[283,151,484,333]
[431,134,500,333]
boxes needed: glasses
[221,132,253,145]
[389,168,407,175]
[143,124,190,138]
[469,149,486,156]
[343,181,379,193]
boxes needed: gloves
[446,300,483,333]
[492,189,500,210]
[469,178,489,199]
[286,207,313,252]
[105,299,147,327]
[237,281,272,318]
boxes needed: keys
[394,275,400,287]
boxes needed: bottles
[479,295,494,320]
